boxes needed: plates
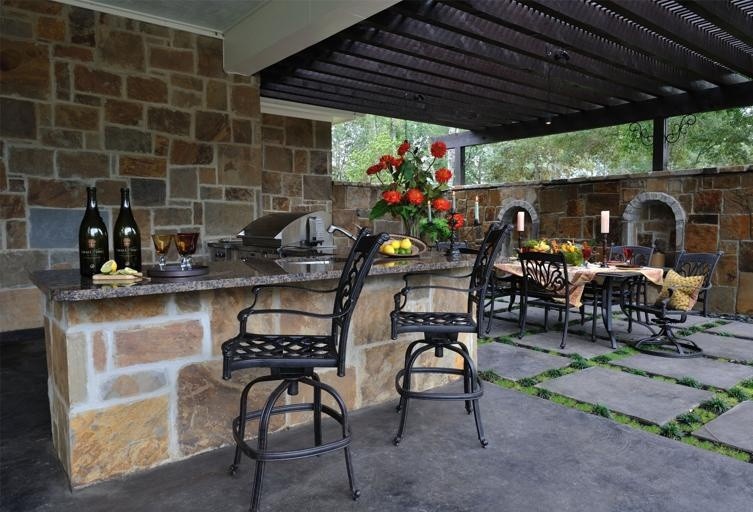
[378,234,427,258]
[148,265,208,277]
[616,264,640,268]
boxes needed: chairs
[622,249,724,359]
[221,226,391,512]
[579,243,655,327]
[390,219,513,448]
[458,220,524,335]
[514,249,600,349]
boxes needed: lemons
[400,238,411,249]
[100,260,117,274]
[391,240,400,249]
[382,245,393,254]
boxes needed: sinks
[273,257,347,274]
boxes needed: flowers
[366,139,465,241]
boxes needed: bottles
[78,187,108,289]
[112,188,141,273]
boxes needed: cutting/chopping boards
[92,273,142,280]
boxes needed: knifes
[134,274,151,281]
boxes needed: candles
[474,196,479,219]
[428,200,432,222]
[452,190,456,209]
[600,210,610,233]
[517,212,525,232]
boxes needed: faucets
[326,224,357,241]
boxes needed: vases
[400,218,423,240]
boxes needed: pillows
[661,269,701,313]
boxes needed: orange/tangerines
[394,248,412,255]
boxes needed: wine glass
[152,234,173,272]
[174,232,199,270]
[623,246,633,265]
